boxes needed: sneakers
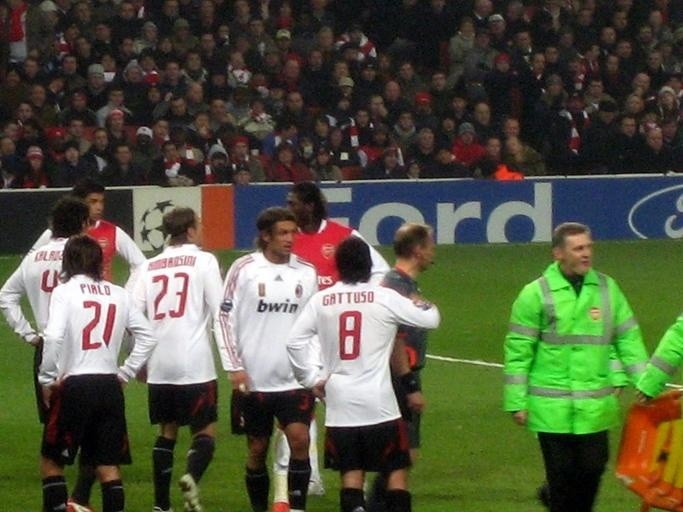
[180,472,203,512]
[66,498,94,512]
[363,482,367,503]
[308,481,325,496]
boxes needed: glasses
[546,79,562,86]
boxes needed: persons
[216,208,318,510]
[636,314,683,404]
[371,223,438,512]
[128,206,246,512]
[284,236,440,512]
[462,2,683,95]
[25,181,148,324]
[0,0,478,121]
[1,103,545,185]
[37,236,158,511]
[272,183,392,511]
[504,223,653,512]
[2,199,133,511]
[523,68,683,174]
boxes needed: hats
[87,64,104,78]
[252,74,266,85]
[288,52,303,65]
[383,144,401,155]
[173,19,189,30]
[362,42,374,55]
[71,89,91,98]
[141,21,157,29]
[136,127,153,140]
[658,86,676,99]
[26,146,45,160]
[43,126,68,138]
[326,114,337,127]
[599,101,618,113]
[54,15,79,34]
[276,29,291,40]
[145,71,159,83]
[61,140,79,151]
[38,0,58,11]
[231,136,248,147]
[569,91,584,99]
[109,109,123,119]
[458,123,476,136]
[336,116,352,125]
[339,42,359,54]
[339,77,355,88]
[217,25,230,33]
[138,47,156,62]
[348,17,362,30]
[415,92,432,105]
[495,54,511,64]
[490,14,504,24]
